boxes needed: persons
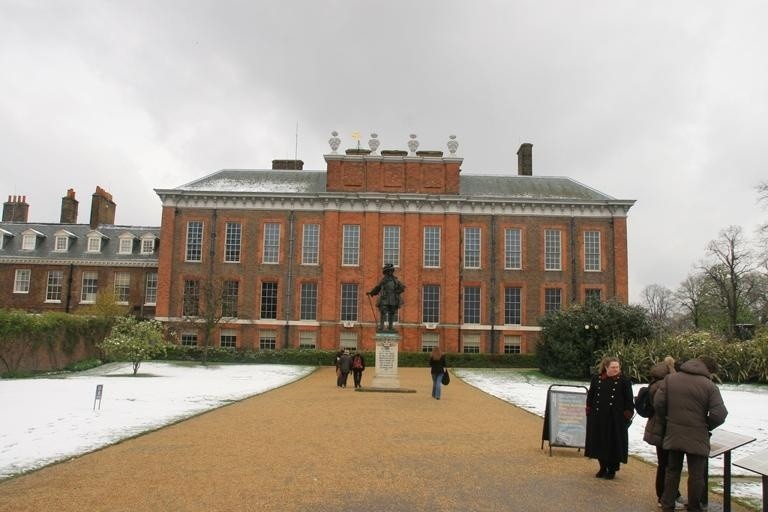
[430,345,446,400]
[366,263,406,331]
[335,349,365,388]
[584,355,728,512]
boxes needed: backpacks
[634,378,661,417]
[353,357,361,369]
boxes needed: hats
[697,355,716,375]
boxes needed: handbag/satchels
[442,370,450,385]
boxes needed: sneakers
[658,496,709,512]
[596,469,615,479]
